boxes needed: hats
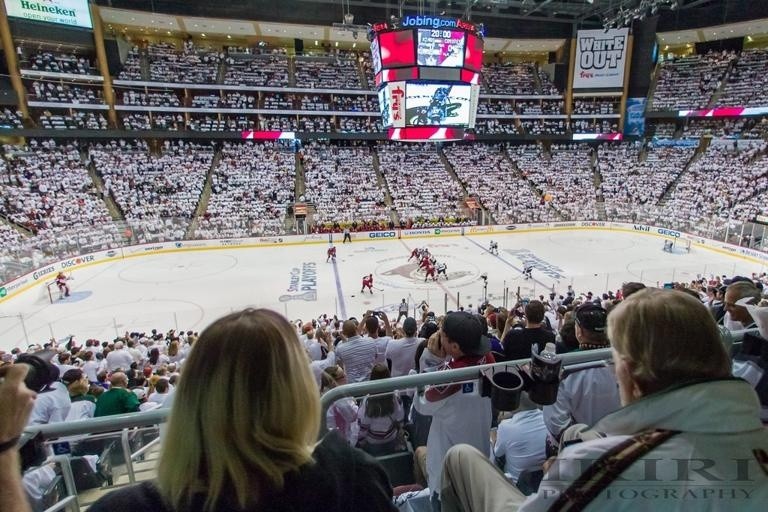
[714,285,727,293]
[446,308,492,356]
[574,301,609,333]
[723,276,753,287]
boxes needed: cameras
[0,347,62,390]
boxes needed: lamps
[344,0,354,24]
[602,0,679,33]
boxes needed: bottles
[540,342,557,373]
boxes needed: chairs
[42,423,160,512]
[375,441,544,512]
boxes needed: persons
[0,34,768,512]
